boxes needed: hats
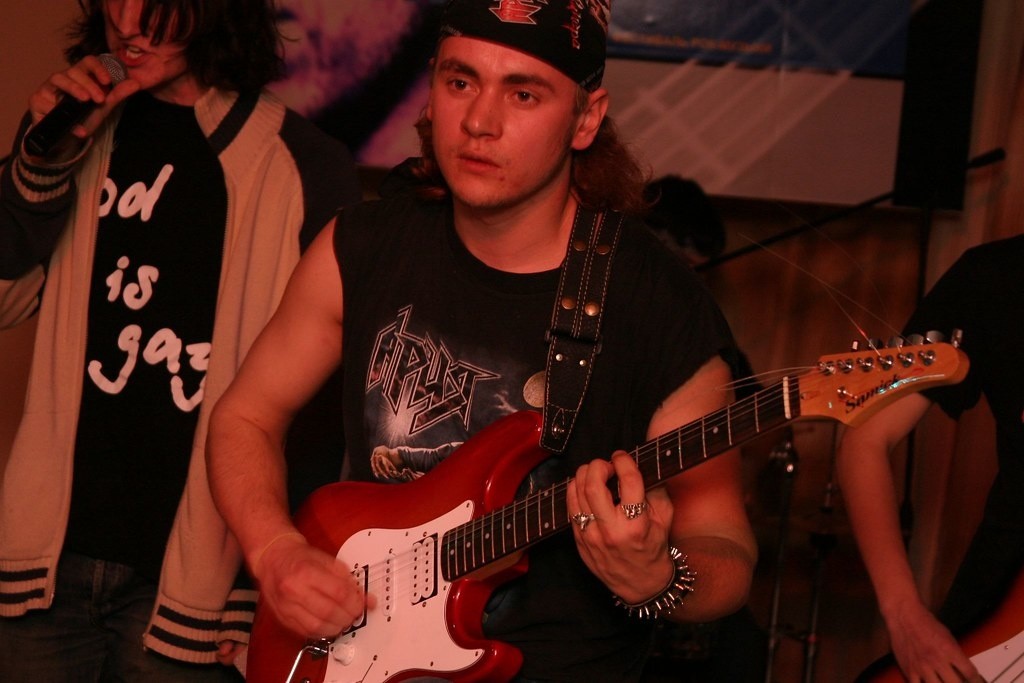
[434,0,612,93]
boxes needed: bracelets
[253,533,306,577]
[613,545,695,620]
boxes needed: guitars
[245,328,971,683]
[870,571,1024,683]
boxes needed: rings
[622,502,647,517]
[572,513,595,529]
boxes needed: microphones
[22,52,128,158]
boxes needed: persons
[2,0,355,683]
[647,172,726,269]
[201,0,757,682]
[837,235,1024,683]
